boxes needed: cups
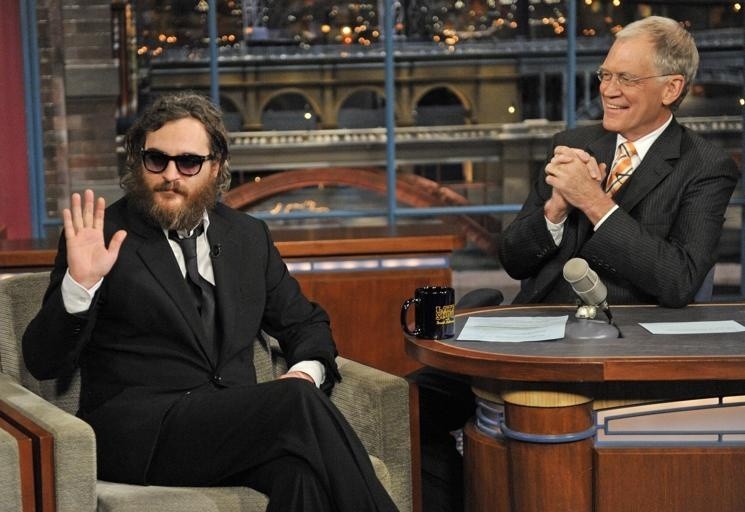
[400,286,455,339]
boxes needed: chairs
[0,415,37,512]
[0,272,422,511]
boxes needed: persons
[499,15,740,307]
[21,88,400,512]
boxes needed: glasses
[139,148,215,177]
[597,67,678,85]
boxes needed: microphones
[562,257,613,321]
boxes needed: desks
[405,299,745,511]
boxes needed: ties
[168,225,217,355]
[604,142,638,197]
